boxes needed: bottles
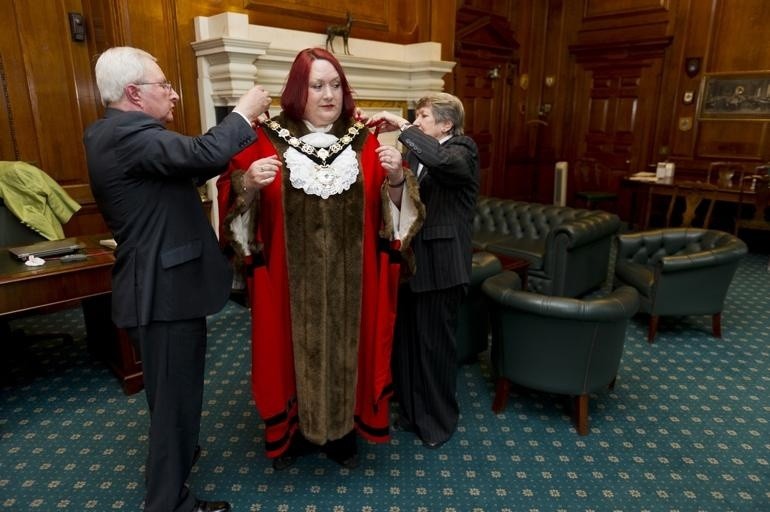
[656,178,674,186]
[655,162,678,178]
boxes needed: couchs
[471,192,620,297]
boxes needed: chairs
[469,245,504,274]
[481,267,642,437]
[618,226,748,345]
[734,175,770,238]
[0,197,58,376]
[666,181,718,229]
[709,163,747,190]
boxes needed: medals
[314,165,341,191]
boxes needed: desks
[0,234,146,399]
[622,168,769,231]
[473,248,536,289]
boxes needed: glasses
[134,79,173,95]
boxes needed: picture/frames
[694,71,769,122]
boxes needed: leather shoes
[191,443,202,469]
[189,497,232,512]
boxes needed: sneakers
[270,435,306,470]
[425,440,441,449]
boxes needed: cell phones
[60,253,88,263]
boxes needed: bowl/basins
[718,170,734,181]
[716,179,733,188]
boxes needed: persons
[216,49,427,468]
[78,46,272,512]
[354,93,480,447]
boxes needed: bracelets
[239,176,253,197]
[388,178,407,188]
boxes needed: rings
[260,166,265,172]
